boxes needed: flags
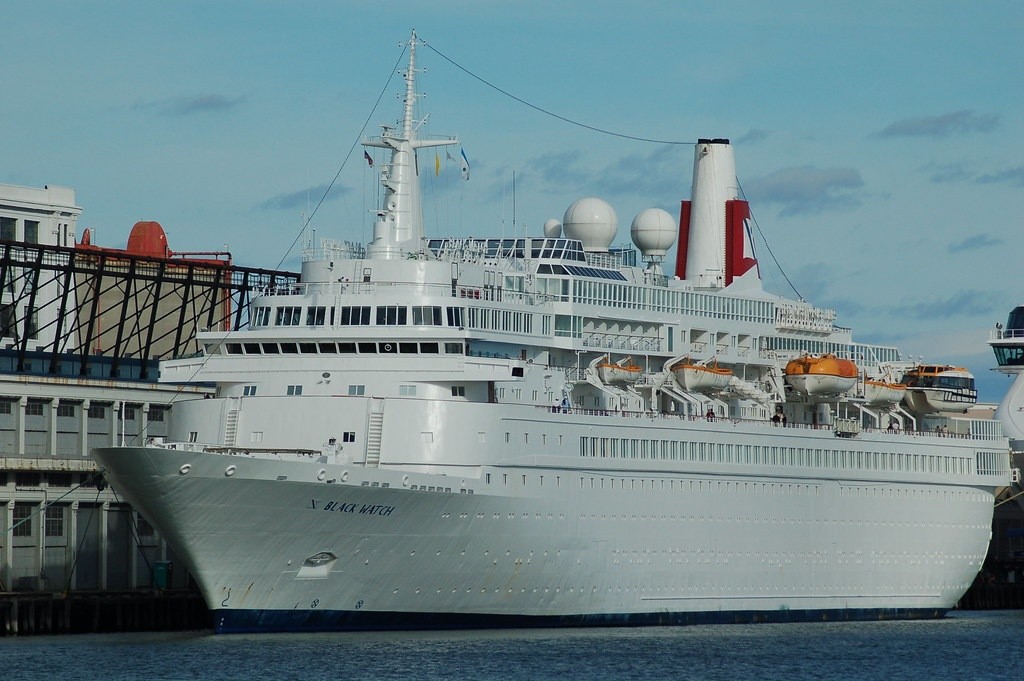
[460,148,470,181]
[435,153,440,176]
[364,151,373,168]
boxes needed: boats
[865,381,907,403]
[785,352,859,396]
[900,365,977,413]
[88,29,1014,635]
[595,364,642,384]
[953,305,1024,610]
[672,356,733,392]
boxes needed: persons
[562,396,570,413]
[554,397,561,413]
[706,409,715,422]
[782,413,787,427]
[934,426,940,437]
[941,425,949,436]
[773,413,780,426]
[888,422,899,434]
